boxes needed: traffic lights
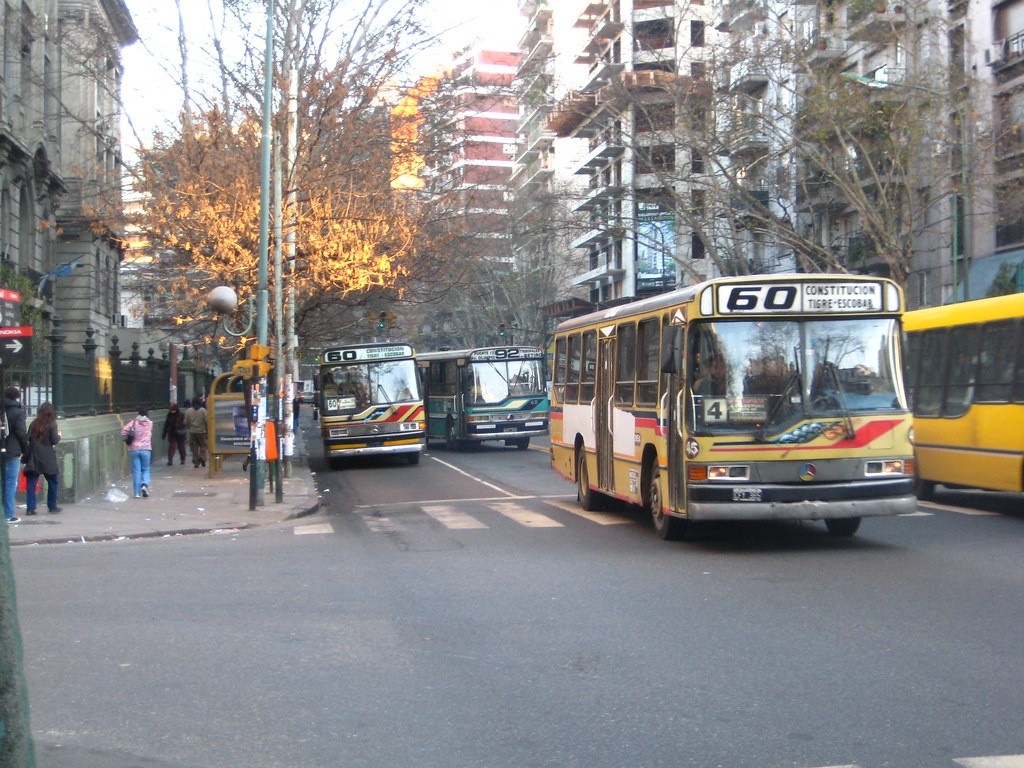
[379,311,386,328]
[389,311,397,330]
[499,322,505,337]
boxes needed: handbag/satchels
[125,418,136,446]
[18,470,40,492]
[175,427,187,437]
[21,443,31,463]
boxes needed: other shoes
[49,506,63,513]
[167,462,172,465]
[27,509,37,515]
[194,463,199,468]
[135,495,140,498]
[243,463,247,471]
[181,461,185,464]
[199,457,206,466]
[142,485,149,497]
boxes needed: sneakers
[6,517,22,524]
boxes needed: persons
[336,373,357,395]
[0,388,30,523]
[663,352,871,394]
[121,409,153,497]
[21,401,63,516]
[160,389,319,468]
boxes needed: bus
[312,341,430,468]
[416,346,551,451]
[899,290,1023,503]
[548,270,917,541]
